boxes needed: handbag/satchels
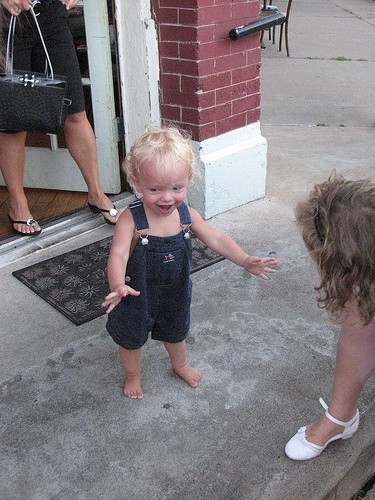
[0,5,71,135]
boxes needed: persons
[0,0,123,235]
[100,128,279,398]
[284,174,374,461]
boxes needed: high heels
[285,398,358,462]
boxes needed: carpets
[12,230,226,326]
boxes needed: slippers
[88,202,116,225]
[7,213,42,235]
